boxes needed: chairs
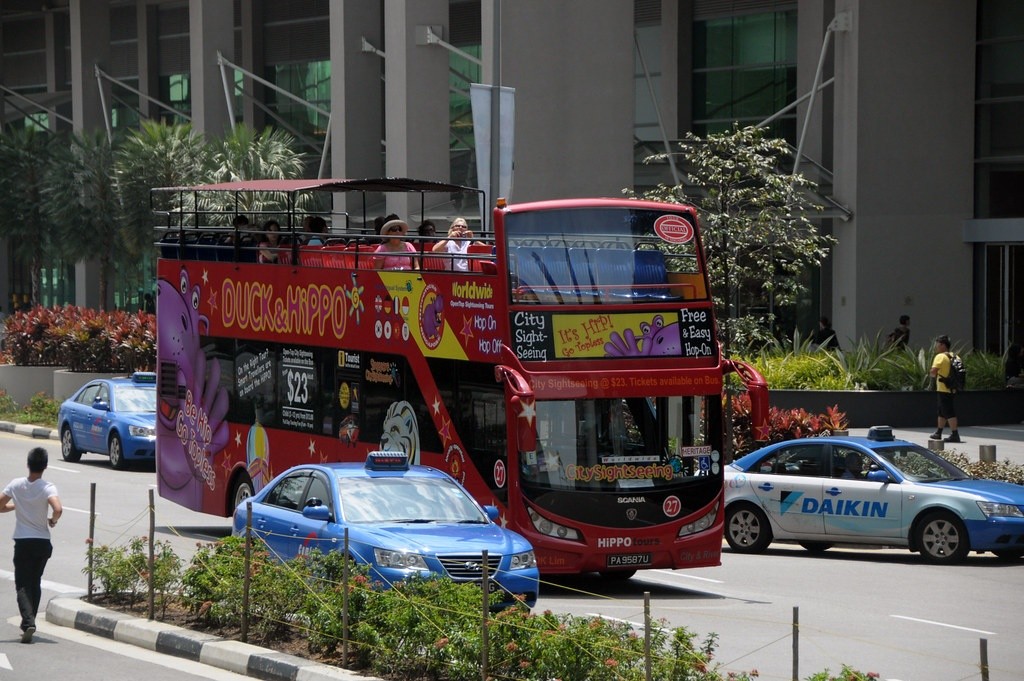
[163,229,684,301]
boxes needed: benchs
[773,460,812,473]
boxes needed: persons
[1005,337,1024,382]
[374,220,420,270]
[433,218,486,270]
[894,316,911,350]
[417,220,437,242]
[842,453,865,479]
[367,213,400,241]
[815,318,840,351]
[226,214,328,264]
[930,335,961,442]
[0,447,62,642]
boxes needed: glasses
[450,224,467,229]
[389,227,403,232]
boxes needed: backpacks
[938,351,967,391]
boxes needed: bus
[151,177,770,589]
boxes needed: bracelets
[50,519,57,524]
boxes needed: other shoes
[20,625,35,644]
[929,431,941,439]
[944,433,960,442]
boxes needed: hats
[380,220,408,241]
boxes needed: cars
[57,371,157,469]
[232,451,540,615]
[41,268,57,295]
[724,425,1024,565]
